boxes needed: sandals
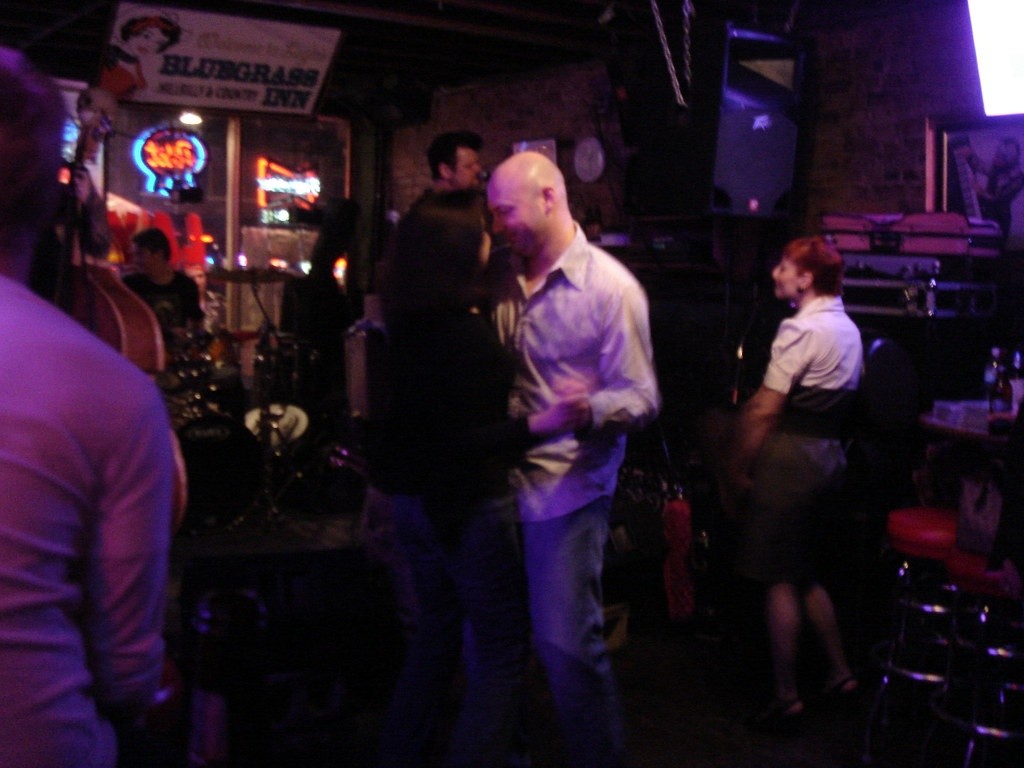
[818,662,870,699]
[768,685,805,726]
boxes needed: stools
[862,507,1024,768]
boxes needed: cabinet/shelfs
[630,214,783,272]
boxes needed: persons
[0,48,180,768]
[123,227,206,362]
[732,233,870,713]
[365,127,662,768]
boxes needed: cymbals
[207,264,281,285]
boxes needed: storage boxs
[789,249,999,320]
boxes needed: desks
[920,411,1008,445]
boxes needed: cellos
[31,105,187,532]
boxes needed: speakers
[698,20,804,218]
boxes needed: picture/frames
[924,112,1024,260]
